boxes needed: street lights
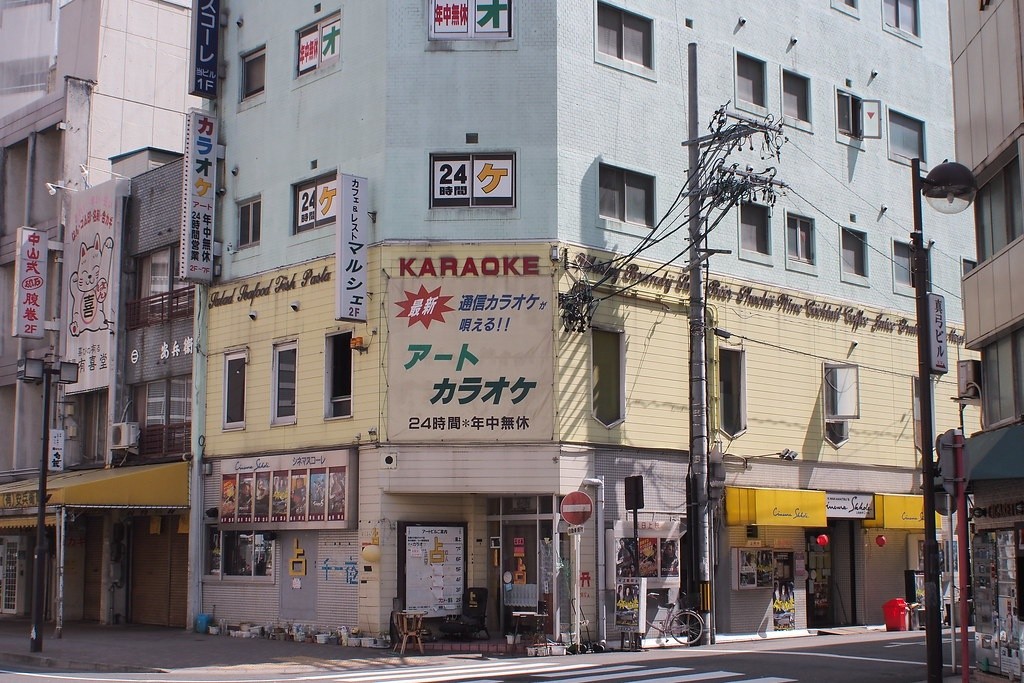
[910,155,978,683]
[17,353,80,654]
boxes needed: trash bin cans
[882,598,907,630]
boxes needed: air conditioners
[957,359,981,399]
[111,422,140,447]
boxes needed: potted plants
[325,630,342,645]
[285,622,293,640]
[208,623,220,635]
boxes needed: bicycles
[628,592,704,648]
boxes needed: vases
[305,637,312,643]
[375,637,386,646]
[280,633,287,640]
[360,638,376,648]
[314,635,330,644]
[294,632,306,642]
[249,625,263,634]
[348,638,361,647]
[274,628,284,640]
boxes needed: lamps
[78,163,131,180]
[205,508,218,517]
[45,183,78,195]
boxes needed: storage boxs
[505,634,522,645]
[230,630,236,637]
[544,646,551,655]
[526,647,546,657]
[235,632,251,638]
[551,646,570,656]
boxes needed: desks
[511,614,549,656]
[391,610,428,656]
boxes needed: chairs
[440,586,491,642]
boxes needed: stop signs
[561,491,594,526]
